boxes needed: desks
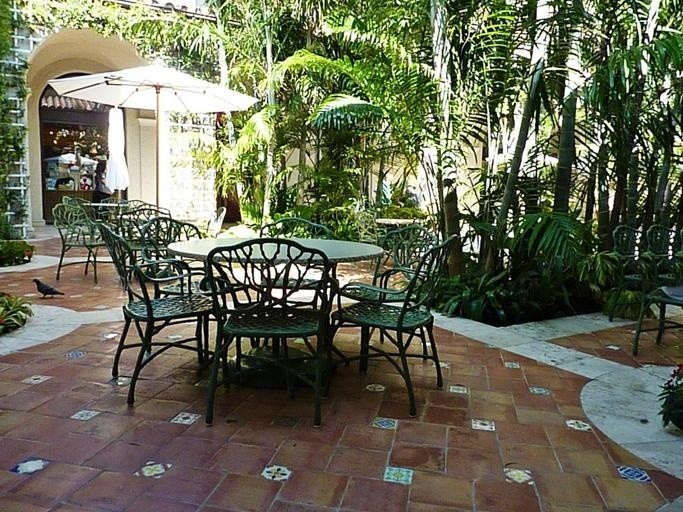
[166,237,387,389]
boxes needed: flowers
[657,362,682,428]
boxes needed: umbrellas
[103,107,130,215]
[47,64,260,273]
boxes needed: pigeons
[31,278,65,300]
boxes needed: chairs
[204,236,331,427]
[50,194,229,295]
[331,225,457,419]
[607,224,683,357]
[95,222,225,409]
[259,216,339,358]
